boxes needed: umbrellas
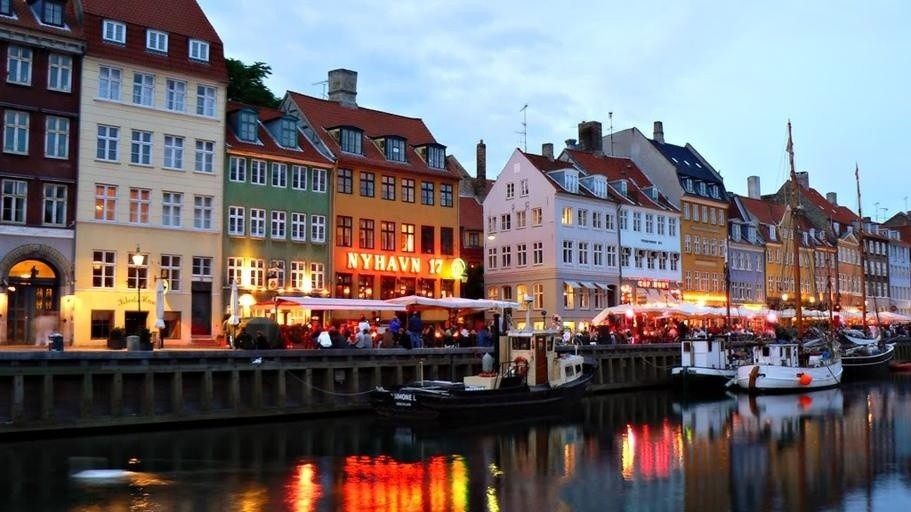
[229,279,240,342]
[155,278,166,347]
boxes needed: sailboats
[669,392,845,508]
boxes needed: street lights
[132,239,144,336]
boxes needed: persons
[303,310,498,349]
[237,327,269,350]
[582,316,721,346]
[823,348,833,365]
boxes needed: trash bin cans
[127,335,140,352]
[49,333,63,351]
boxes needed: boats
[369,296,599,421]
